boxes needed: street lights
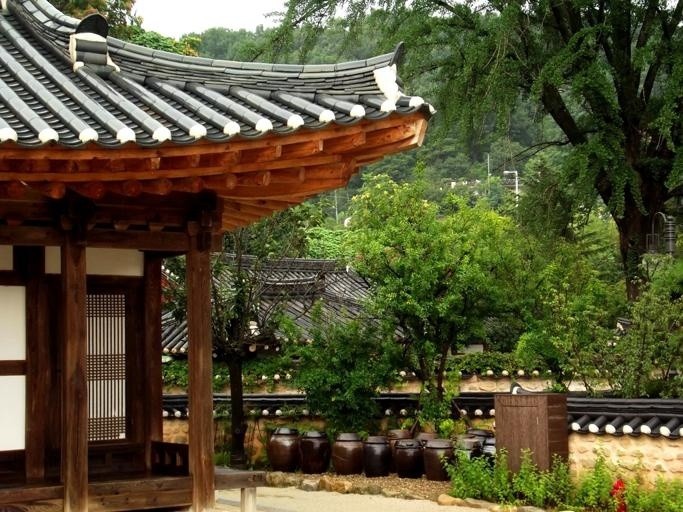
[503,170,518,210]
